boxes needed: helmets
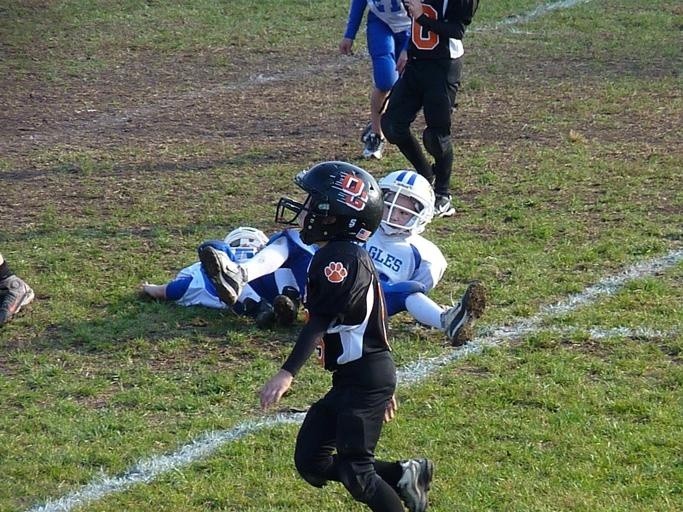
[379,171,436,242]
[275,160,384,246]
[224,227,269,262]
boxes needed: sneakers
[361,120,386,160]
[396,459,434,512]
[256,298,297,329]
[201,246,248,305]
[0,275,34,329]
[434,192,455,221]
[446,281,487,347]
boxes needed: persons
[0,252,35,324]
[339,0,410,161]
[381,0,478,216]
[261,160,434,511]
[200,170,487,348]
[139,224,298,329]
